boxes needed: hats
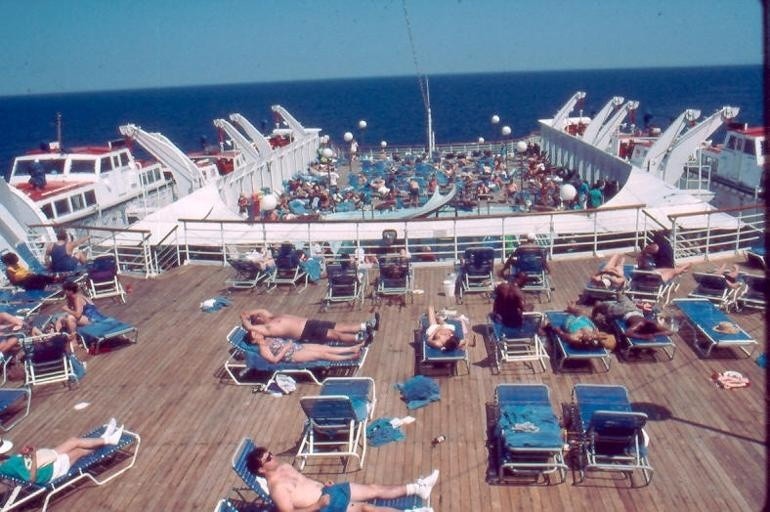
[0,438,14,453]
[713,321,739,334]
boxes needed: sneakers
[417,469,440,499]
[103,417,117,435]
[358,325,373,344]
[371,312,380,330]
[108,424,124,445]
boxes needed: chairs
[0,388,32,432]
[2,241,138,390]
[0,423,141,512]
[494,383,569,484]
[293,378,375,472]
[232,436,428,512]
[572,383,654,485]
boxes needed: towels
[710,370,749,391]
[390,415,415,427]
[393,375,442,410]
[200,295,229,314]
[363,418,405,446]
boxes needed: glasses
[262,451,272,463]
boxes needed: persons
[0,231,97,363]
[243,330,366,365]
[241,230,691,296]
[0,418,124,486]
[248,447,440,512]
[489,271,533,328]
[552,301,600,350]
[426,305,466,352]
[239,138,606,223]
[592,292,673,340]
[240,308,380,344]
[717,263,740,288]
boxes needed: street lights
[479,137,484,146]
[502,126,511,177]
[359,120,367,154]
[491,116,500,161]
[344,132,353,172]
[380,141,386,150]
[560,184,576,208]
[322,148,333,197]
[517,141,527,191]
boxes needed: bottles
[432,435,446,445]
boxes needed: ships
[0,119,298,260]
[576,112,767,248]
